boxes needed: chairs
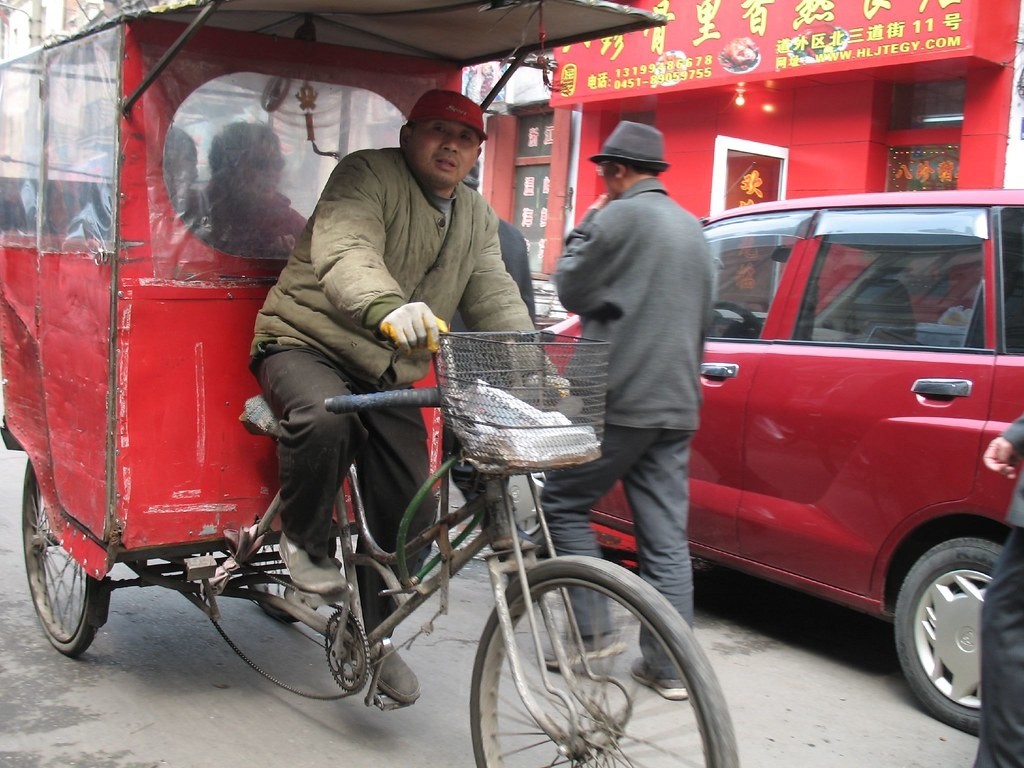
[812,279,916,344]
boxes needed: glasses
[238,158,286,171]
[596,160,615,176]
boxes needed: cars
[525,187,1024,738]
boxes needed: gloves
[377,301,449,359]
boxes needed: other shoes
[542,630,626,669]
[279,531,347,596]
[367,640,422,706]
[630,655,689,702]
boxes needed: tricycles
[0,0,742,768]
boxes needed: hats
[587,119,670,175]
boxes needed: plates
[718,42,761,74]
[655,51,687,87]
[788,26,849,63]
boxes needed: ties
[409,88,489,146]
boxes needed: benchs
[0,177,203,241]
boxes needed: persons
[249,87,571,704]
[969,415,1024,768]
[540,122,715,703]
[166,121,537,562]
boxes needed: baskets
[430,322,610,471]
